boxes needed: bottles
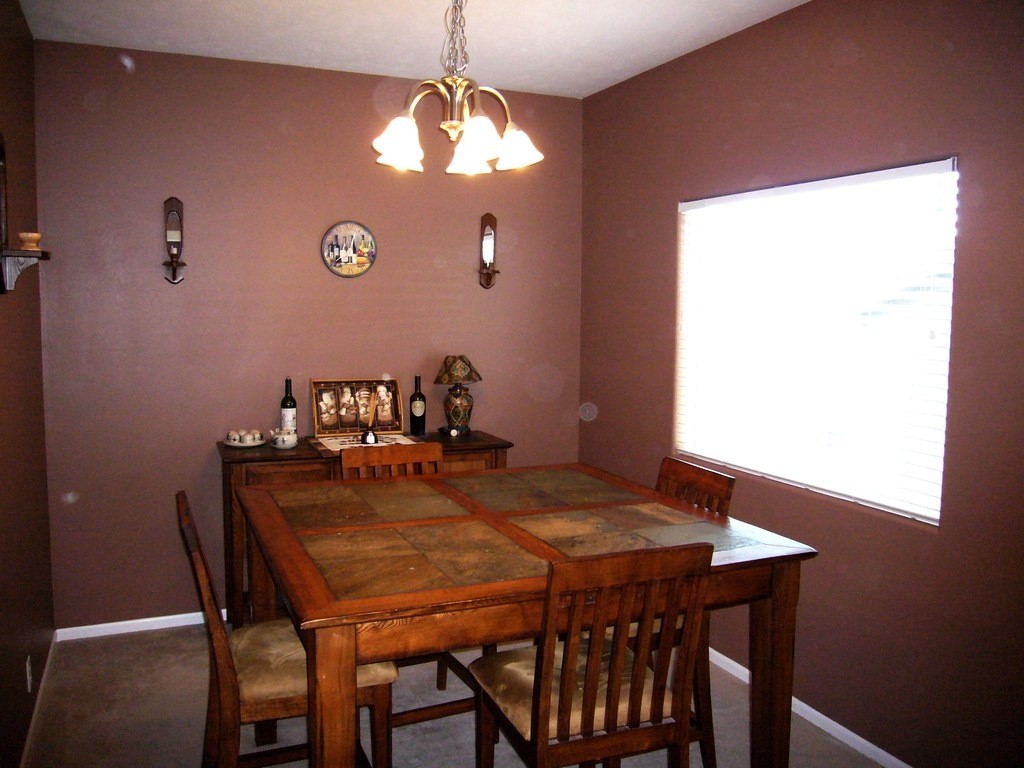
[281,375,297,435]
[362,426,378,444]
[328,235,373,263]
[410,373,426,436]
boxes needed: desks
[217,431,523,631]
[239,462,818,767]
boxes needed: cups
[227,429,263,443]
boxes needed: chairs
[559,455,736,727]
[469,537,715,768]
[177,491,399,768]
[338,442,450,691]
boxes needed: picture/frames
[320,221,378,278]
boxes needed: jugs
[270,428,298,448]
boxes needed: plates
[222,436,268,447]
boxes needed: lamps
[433,355,484,437]
[478,213,500,289]
[371,0,544,177]
[164,196,187,283]
